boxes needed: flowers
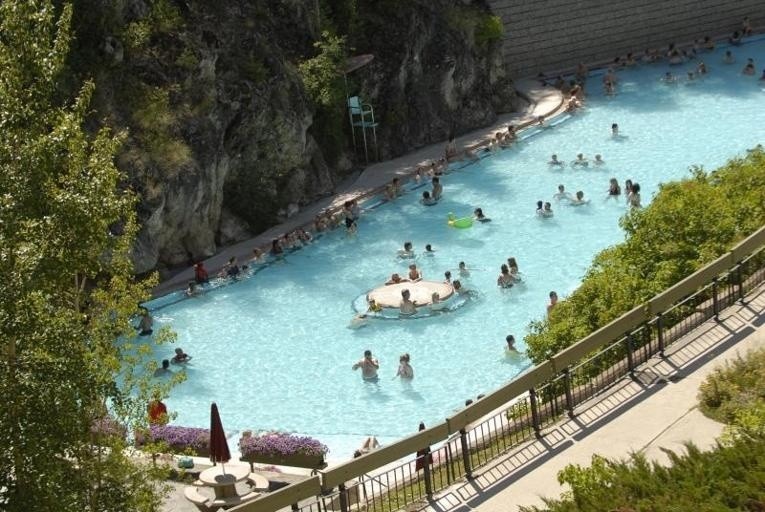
[142,426,212,449]
[236,427,331,460]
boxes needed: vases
[170,443,211,457]
[243,451,328,471]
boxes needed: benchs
[183,473,270,508]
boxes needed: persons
[488,61,593,154]
[474,122,642,289]
[186,136,468,296]
[369,280,461,314]
[351,349,413,378]
[505,291,566,349]
[133,307,192,424]
[603,16,754,92]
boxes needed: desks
[198,463,251,498]
